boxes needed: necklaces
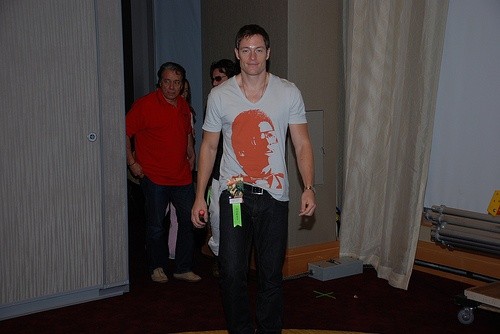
[240,73,266,102]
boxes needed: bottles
[193,209,208,246]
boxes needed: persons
[125,62,202,281]
[210,59,239,277]
[191,24,316,334]
[153,80,195,260]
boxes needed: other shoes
[173,271,202,282]
[151,266,168,282]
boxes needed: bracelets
[129,161,136,166]
[303,184,315,193]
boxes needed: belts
[243,183,269,195]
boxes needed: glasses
[212,76,226,82]
